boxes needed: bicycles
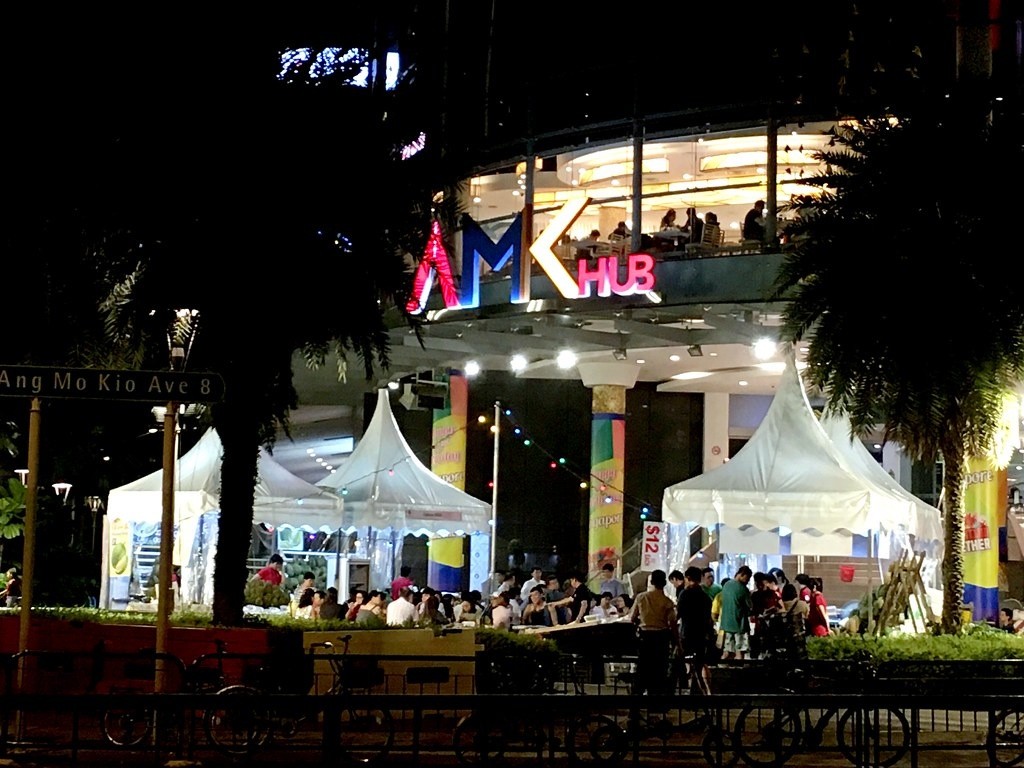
[732,652,911,768]
[100,651,228,749]
[203,634,396,764]
[985,707,1024,768]
[449,655,626,768]
[588,647,743,768]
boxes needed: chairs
[560,213,788,260]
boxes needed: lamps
[687,344,703,356]
[613,349,627,361]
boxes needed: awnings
[311,388,491,602]
[656,331,950,641]
[97,421,344,609]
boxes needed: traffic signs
[0,363,226,405]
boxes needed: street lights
[151,308,201,693]
[52,482,73,507]
[83,494,102,552]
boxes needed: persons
[579,204,765,259]
[246,554,834,707]
